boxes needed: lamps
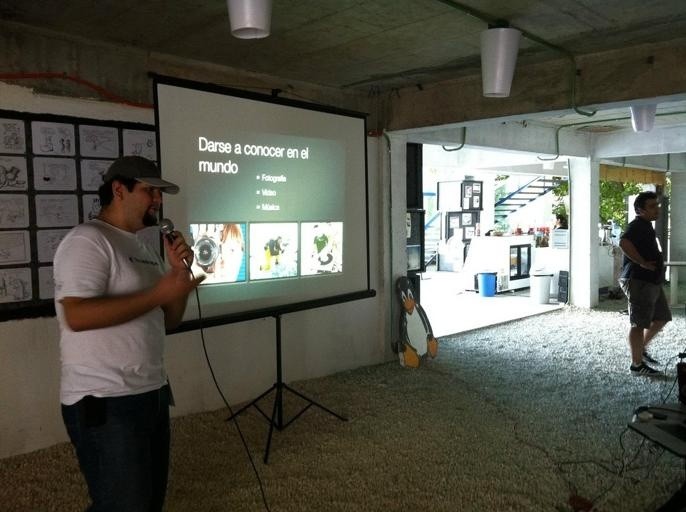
[479,19,522,98]
[225,1,274,42]
[629,101,658,133]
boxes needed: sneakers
[629,361,660,377]
[640,351,660,366]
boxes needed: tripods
[224,312,349,464]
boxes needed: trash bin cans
[529,272,553,304]
[478,272,497,297]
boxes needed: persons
[616,192,674,376]
[555,216,566,229]
[52,157,206,511]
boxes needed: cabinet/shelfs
[436,179,495,272]
[453,234,535,293]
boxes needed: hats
[101,156,179,194]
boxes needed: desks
[626,402,686,460]
[664,261,685,310]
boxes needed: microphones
[159,218,190,268]
